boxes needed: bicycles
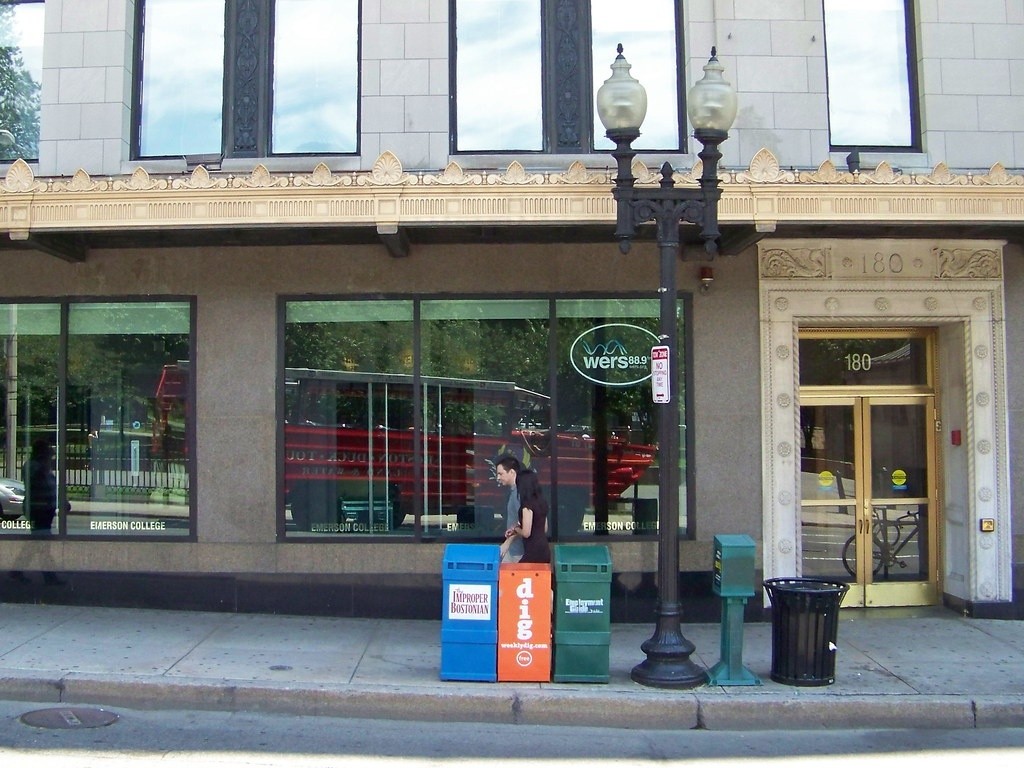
[842,505,920,576]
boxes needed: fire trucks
[151,360,656,531]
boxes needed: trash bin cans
[761,577,851,687]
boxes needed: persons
[496,457,551,563]
[21,439,72,534]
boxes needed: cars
[0,477,25,521]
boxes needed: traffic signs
[651,346,670,404]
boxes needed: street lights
[596,43,738,690]
